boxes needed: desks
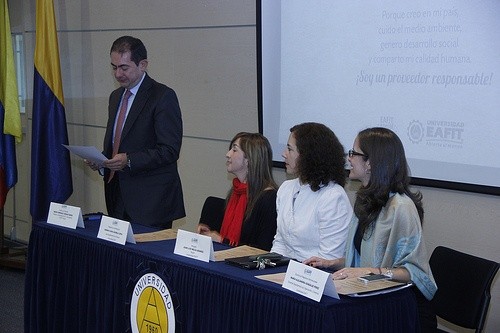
[23,212,430,333]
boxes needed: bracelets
[377,267,382,275]
[385,267,392,280]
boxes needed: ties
[110,90,133,177]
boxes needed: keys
[253,257,276,270]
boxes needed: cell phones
[358,274,392,283]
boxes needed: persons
[196,132,279,250]
[303,127,438,333]
[82,36,187,229]
[270,122,353,261]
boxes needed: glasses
[349,149,363,157]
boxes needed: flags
[30,0,73,220]
[0,0,22,205]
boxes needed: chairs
[431,244,499,332]
[198,196,225,232]
